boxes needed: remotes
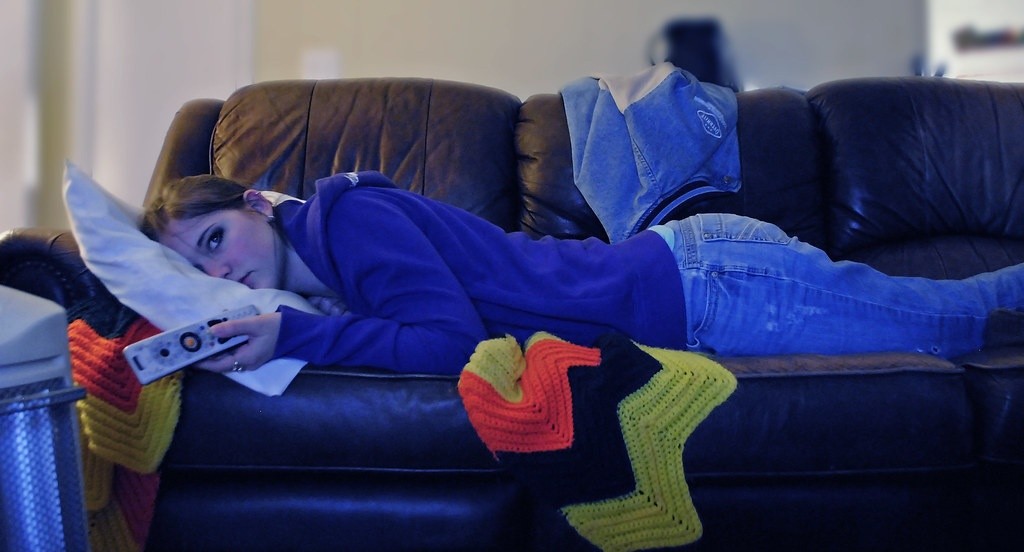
[121,305,262,385]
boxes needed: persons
[142,169,1023,377]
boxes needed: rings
[234,361,243,373]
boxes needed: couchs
[3,74,1024,548]
